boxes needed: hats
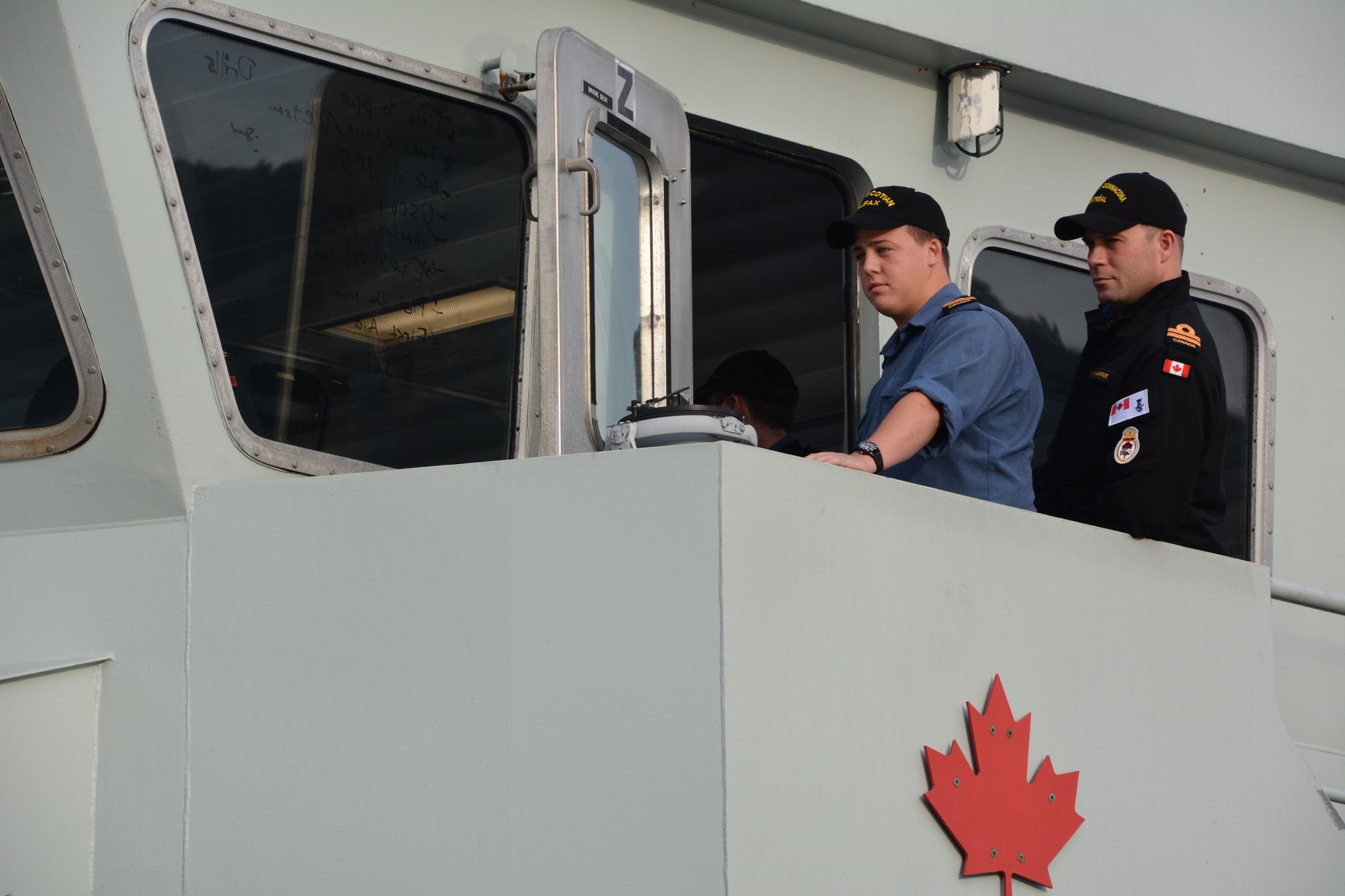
[694,349,797,410]
[825,186,950,251]
[1054,172,1187,241]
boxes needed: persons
[694,349,822,458]
[805,187,1043,512]
[1030,172,1227,556]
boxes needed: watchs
[852,440,883,476]
